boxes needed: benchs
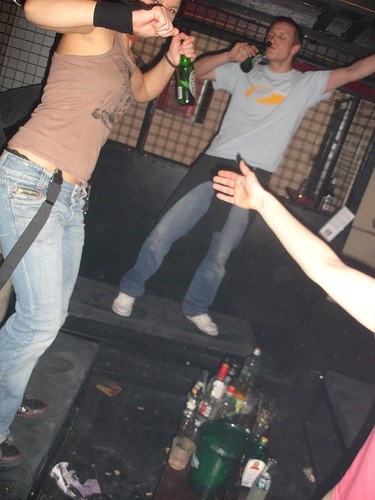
[0,83,375,500]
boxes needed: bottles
[246,457,278,500]
[231,436,271,500]
[319,176,337,214]
[240,41,272,73]
[177,346,277,442]
[176,39,196,106]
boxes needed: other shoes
[16,394,48,419]
[185,313,219,336]
[111,292,136,317]
[0,442,21,467]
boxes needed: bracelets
[93,1,134,36]
[165,55,176,68]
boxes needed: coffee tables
[26,364,271,500]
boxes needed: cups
[167,435,197,471]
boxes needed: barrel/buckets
[190,419,256,500]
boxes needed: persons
[111,16,375,336]
[212,159,375,500]
[0,0,196,469]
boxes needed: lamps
[316,8,369,42]
